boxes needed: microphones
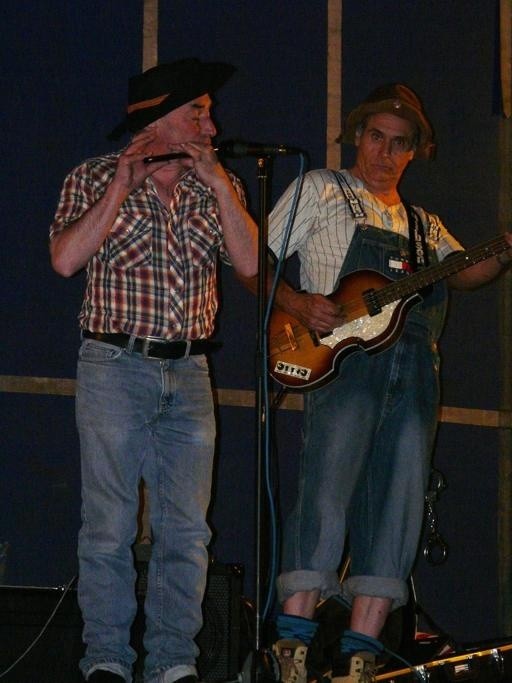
[216,136,300,166]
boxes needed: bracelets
[495,250,511,266]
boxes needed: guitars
[265,232,511,391]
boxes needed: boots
[330,649,376,683]
[268,637,309,683]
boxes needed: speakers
[129,543,244,683]
[0,583,85,681]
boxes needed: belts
[81,327,226,361]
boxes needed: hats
[104,54,232,145]
[334,82,435,161]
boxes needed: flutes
[142,144,229,163]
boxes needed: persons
[236,76,512,682]
[46,59,259,682]
